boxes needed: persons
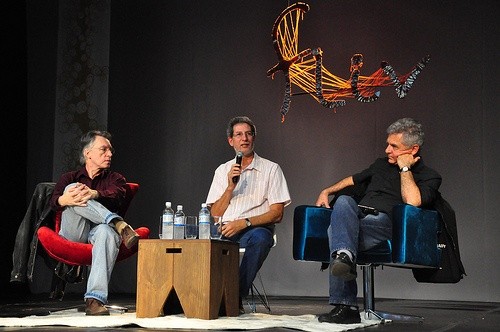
[205,116,292,314]
[315,118,441,324]
[50,130,139,315]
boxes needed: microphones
[357,205,379,215]
[233,151,243,184]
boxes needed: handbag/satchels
[412,235,463,284]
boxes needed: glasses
[232,131,255,137]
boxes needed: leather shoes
[330,252,357,281]
[85,297,110,315]
[318,304,361,324]
[120,224,139,249]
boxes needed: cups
[185,216,197,239]
[210,216,222,239]
[159,216,162,239]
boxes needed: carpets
[0,305,381,332]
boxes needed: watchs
[244,218,253,228]
[399,166,412,172]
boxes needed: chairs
[293,198,444,321]
[37,182,151,302]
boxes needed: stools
[239,233,278,313]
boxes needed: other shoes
[239,298,245,313]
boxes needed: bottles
[174,205,185,239]
[162,202,174,239]
[199,203,210,239]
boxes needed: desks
[135,239,240,320]
